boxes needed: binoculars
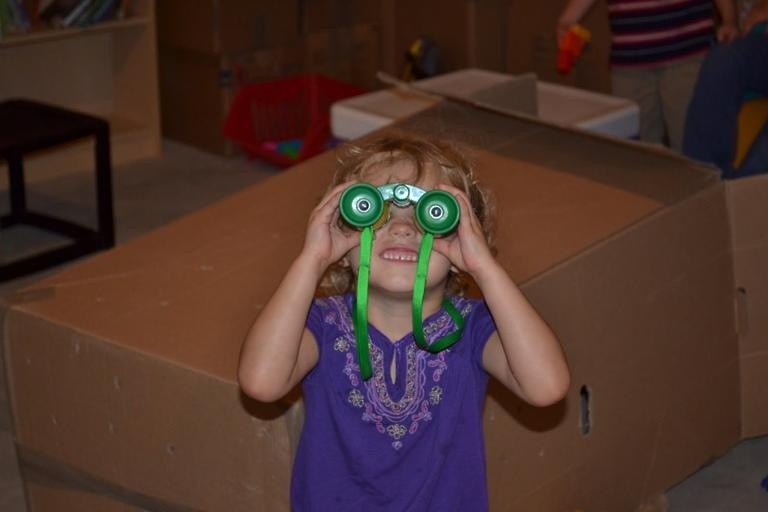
[339,183,460,239]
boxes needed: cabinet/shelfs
[0,0,162,191]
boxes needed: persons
[236,125,571,512]
[682,0,768,180]
[559,0,740,154]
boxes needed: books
[0,0,132,35]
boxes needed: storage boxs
[0,71,768,512]
